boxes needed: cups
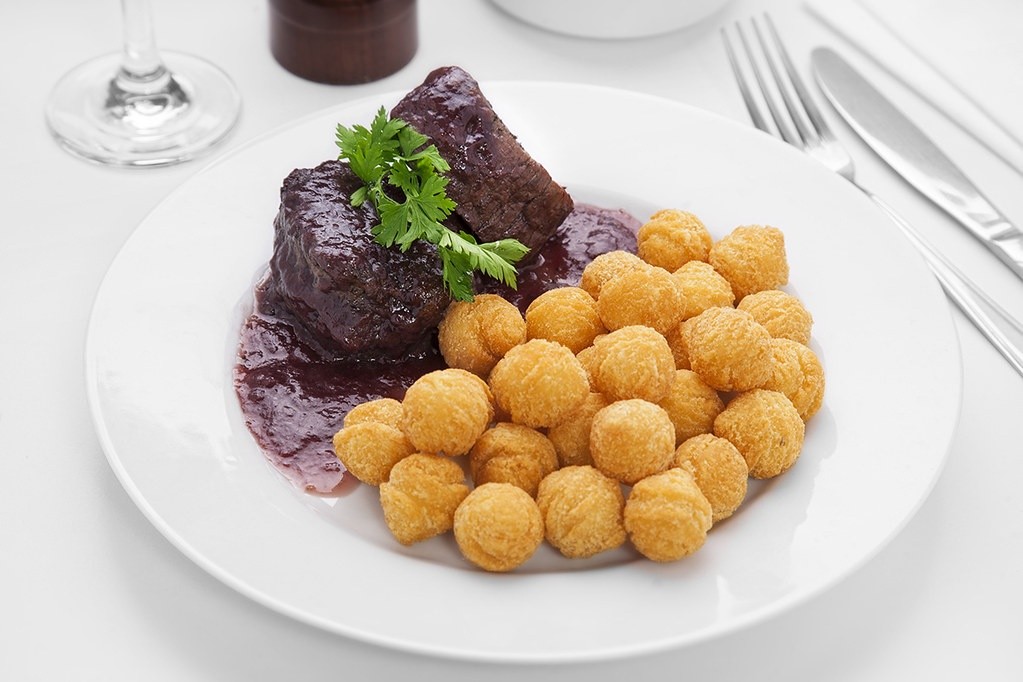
[267,0,419,85]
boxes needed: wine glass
[46,0,241,170]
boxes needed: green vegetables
[332,106,532,304]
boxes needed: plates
[492,0,725,38]
[85,82,965,664]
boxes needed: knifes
[811,47,1023,279]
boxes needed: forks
[721,12,1023,376]
